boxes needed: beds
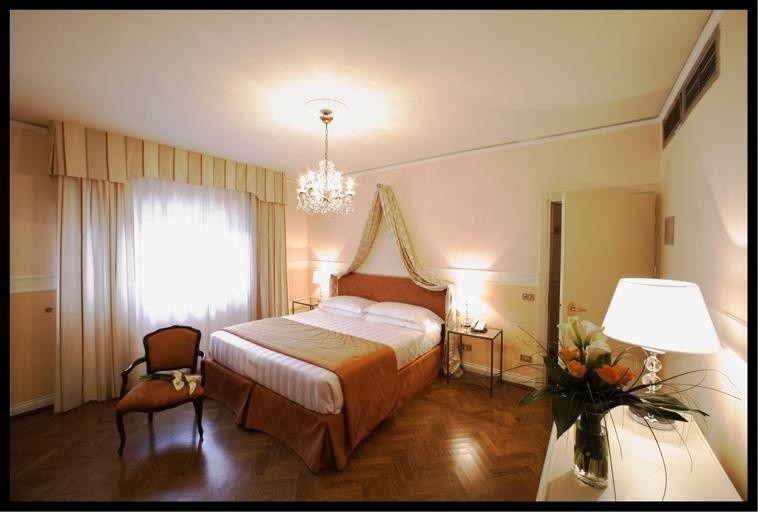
[202,309,445,475]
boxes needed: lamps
[597,276,724,433]
[294,98,355,214]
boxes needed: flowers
[136,367,205,395]
[488,311,739,503]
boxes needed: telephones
[470,320,487,331]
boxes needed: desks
[448,326,503,395]
[292,299,322,313]
[537,399,744,503]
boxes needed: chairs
[115,324,209,457]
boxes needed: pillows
[319,295,444,336]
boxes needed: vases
[572,403,611,489]
